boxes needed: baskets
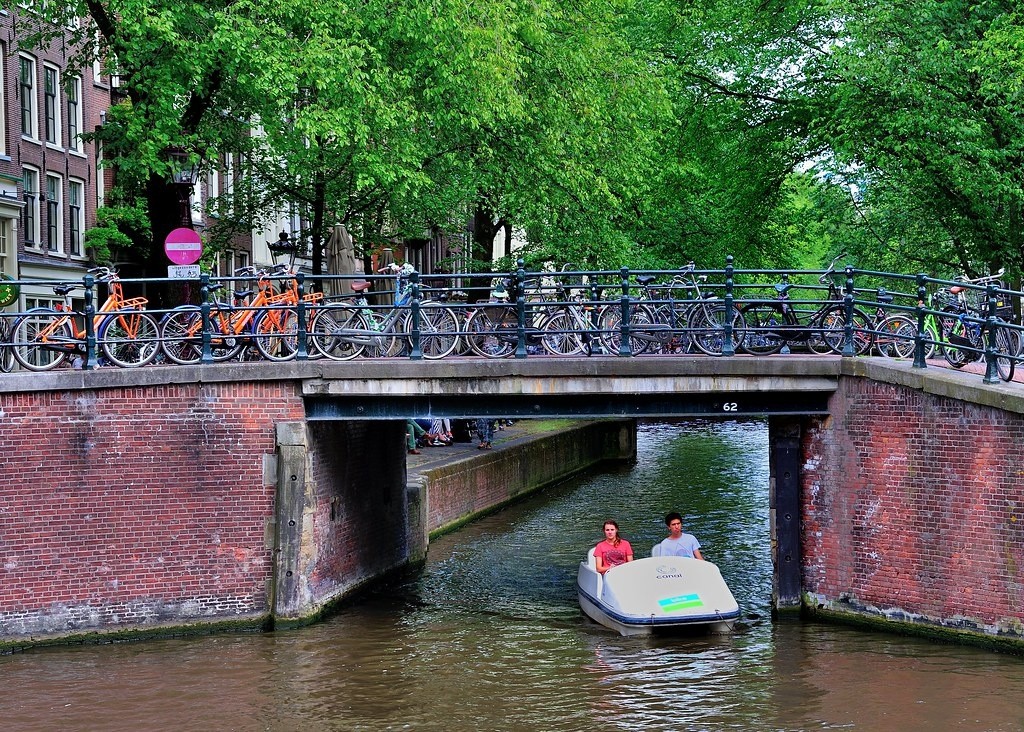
[976,281,1014,320]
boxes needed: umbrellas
[325,223,355,358]
[377,249,396,319]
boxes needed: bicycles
[0,264,1024,383]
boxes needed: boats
[577,547,741,636]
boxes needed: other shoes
[408,449,421,454]
[426,441,432,447]
[433,431,453,445]
[499,426,504,431]
[423,433,438,440]
[478,441,487,448]
[487,442,492,450]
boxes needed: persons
[657,513,704,560]
[498,419,514,430]
[407,419,453,454]
[477,419,496,449]
[593,520,633,574]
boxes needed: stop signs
[164,228,204,263]
